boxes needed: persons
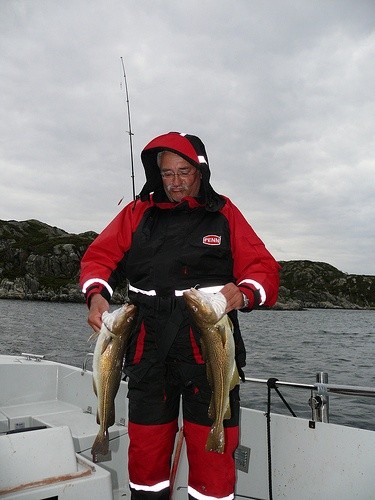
[78,129,283,500]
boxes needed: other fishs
[182,283,241,455]
[90,302,139,455]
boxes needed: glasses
[160,169,198,178]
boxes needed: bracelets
[240,290,249,308]
